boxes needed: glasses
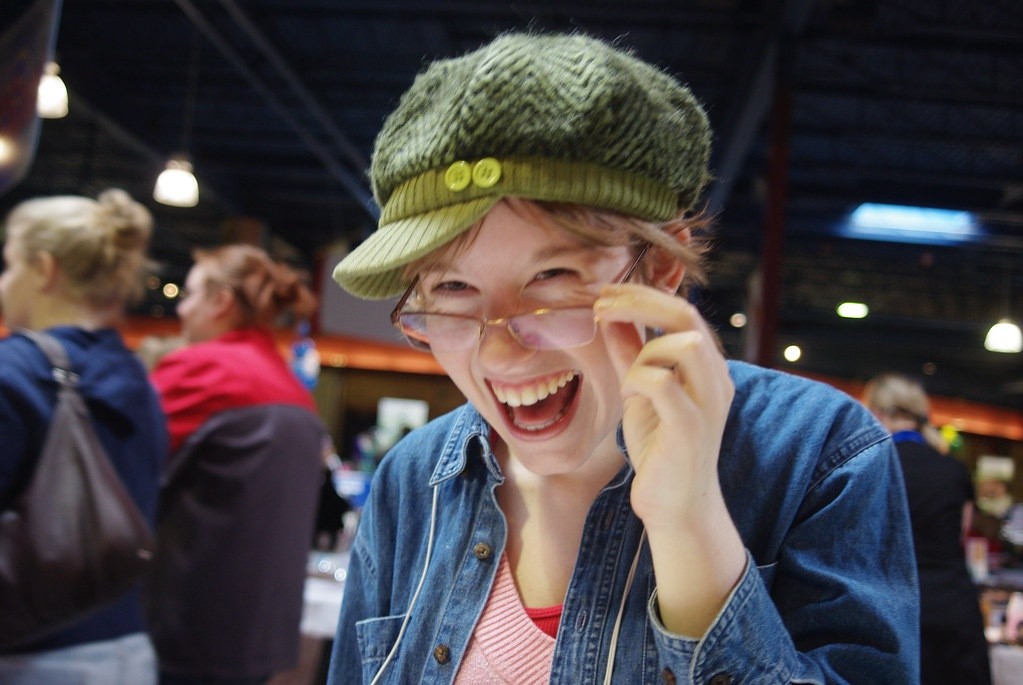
[389,241,651,353]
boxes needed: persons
[0,188,169,685]
[326,32,921,685]
[866,373,1023,685]
[146,243,325,685]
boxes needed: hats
[333,33,709,299]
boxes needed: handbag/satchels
[0,325,160,655]
